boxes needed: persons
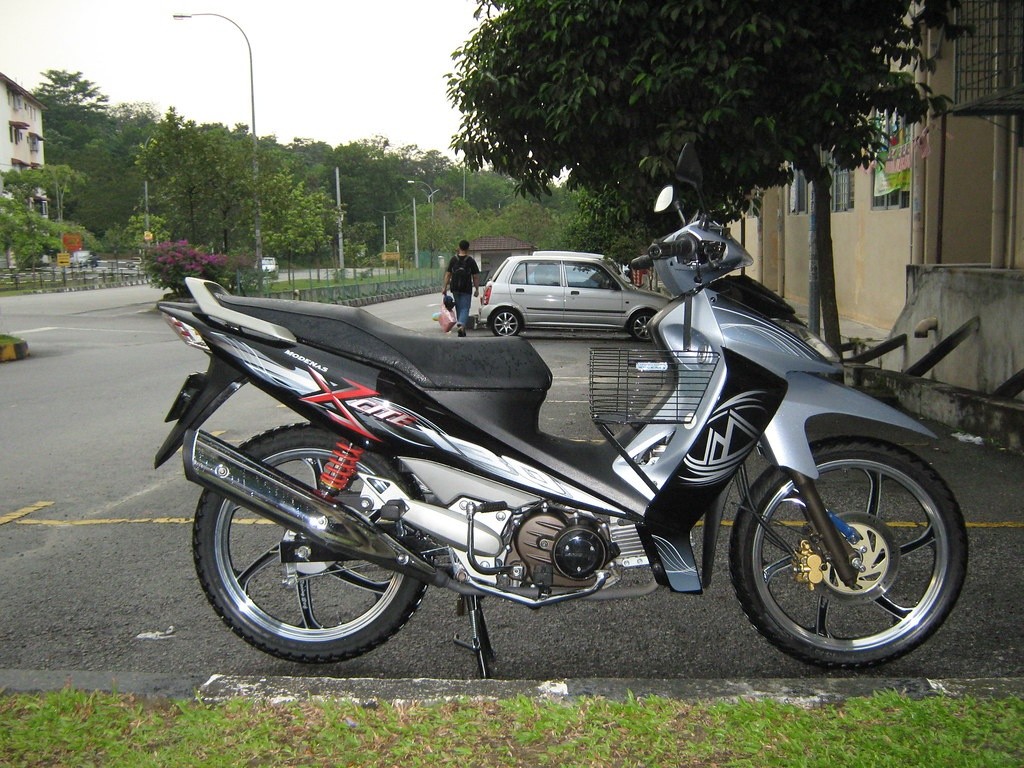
[441,240,479,337]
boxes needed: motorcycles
[152,142,970,680]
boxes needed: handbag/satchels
[443,294,455,310]
[439,295,457,333]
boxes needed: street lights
[172,11,264,292]
[420,188,441,203]
[406,179,434,270]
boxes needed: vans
[532,250,633,288]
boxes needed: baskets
[588,347,720,423]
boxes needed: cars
[69,251,98,268]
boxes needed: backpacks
[450,254,472,293]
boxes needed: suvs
[477,255,674,342]
[253,257,280,280]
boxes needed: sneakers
[457,325,466,337]
